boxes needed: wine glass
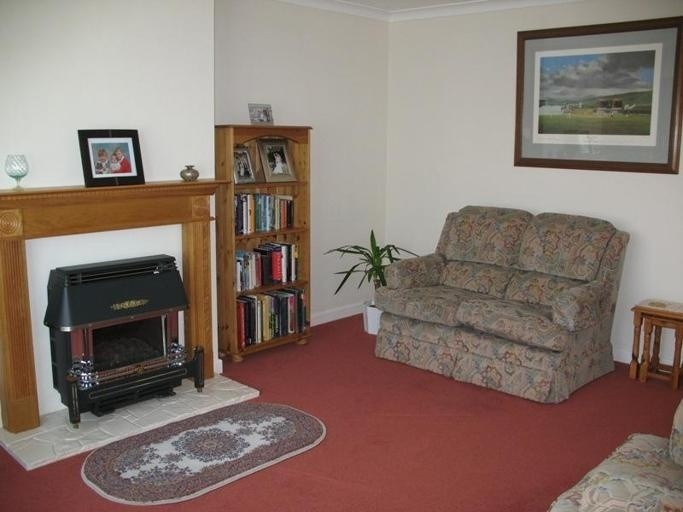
[5,153,29,191]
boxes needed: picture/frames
[256,138,298,182]
[514,16,683,175]
[232,147,255,184]
[77,128,146,188]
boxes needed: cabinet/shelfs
[214,123,312,362]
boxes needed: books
[236,238,302,290]
[236,284,306,349]
[234,193,294,235]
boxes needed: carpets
[81,403,326,506]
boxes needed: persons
[238,157,251,177]
[95,146,131,174]
[272,150,284,174]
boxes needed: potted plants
[322,229,420,336]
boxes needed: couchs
[549,401,683,512]
[373,205,630,404]
